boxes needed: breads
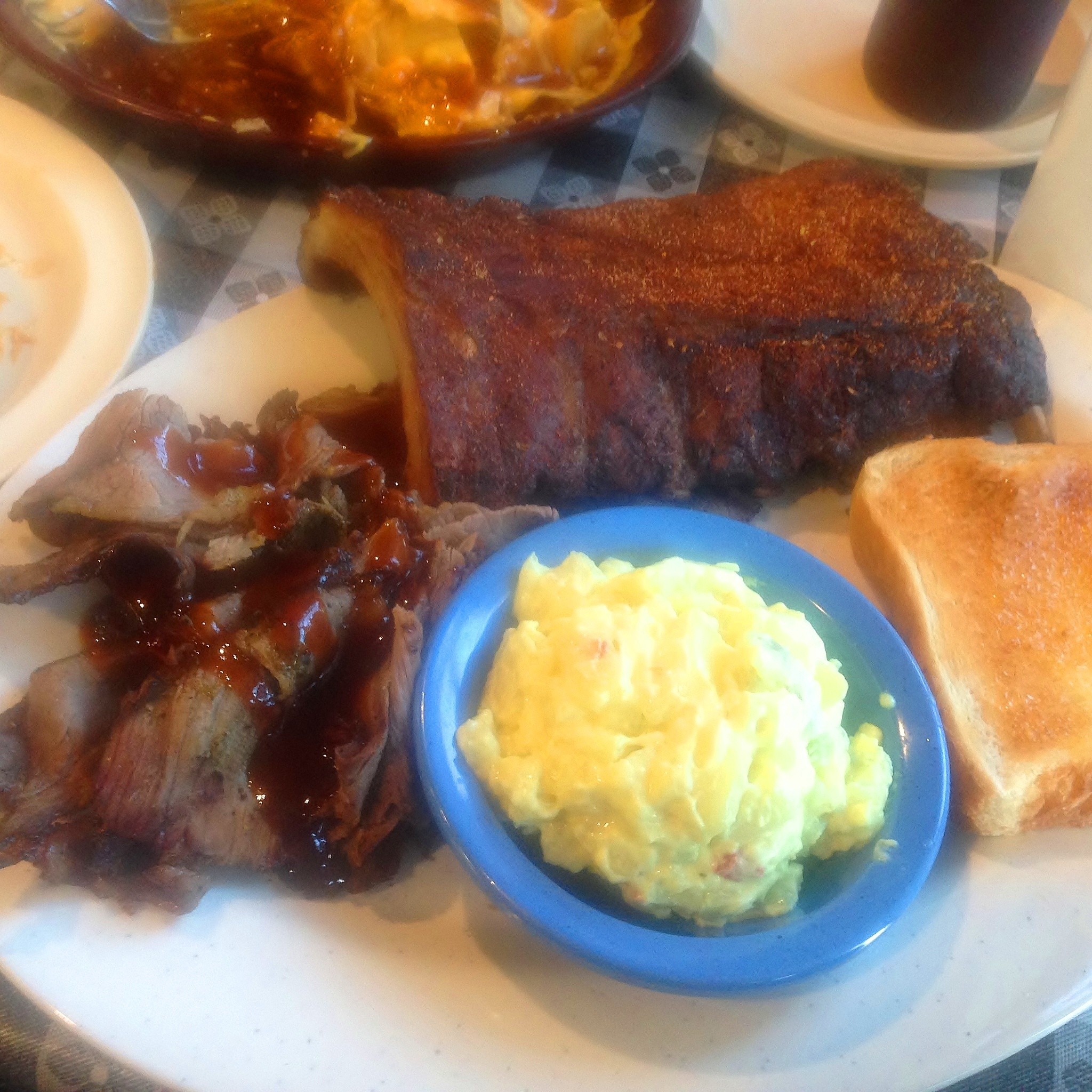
[846,434,1092,838]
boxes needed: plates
[415,500,954,984]
[680,0,1092,183]
[0,0,705,167]
[0,230,1091,1092]
[1,88,157,502]
